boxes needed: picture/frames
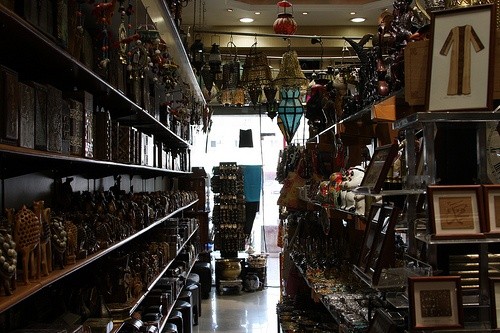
[482,184,500,238]
[488,277,500,329]
[407,276,465,331]
[428,184,486,239]
[425,3,497,110]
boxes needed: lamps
[186,0,311,143]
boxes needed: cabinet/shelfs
[275,0,500,333]
[0,0,210,333]
